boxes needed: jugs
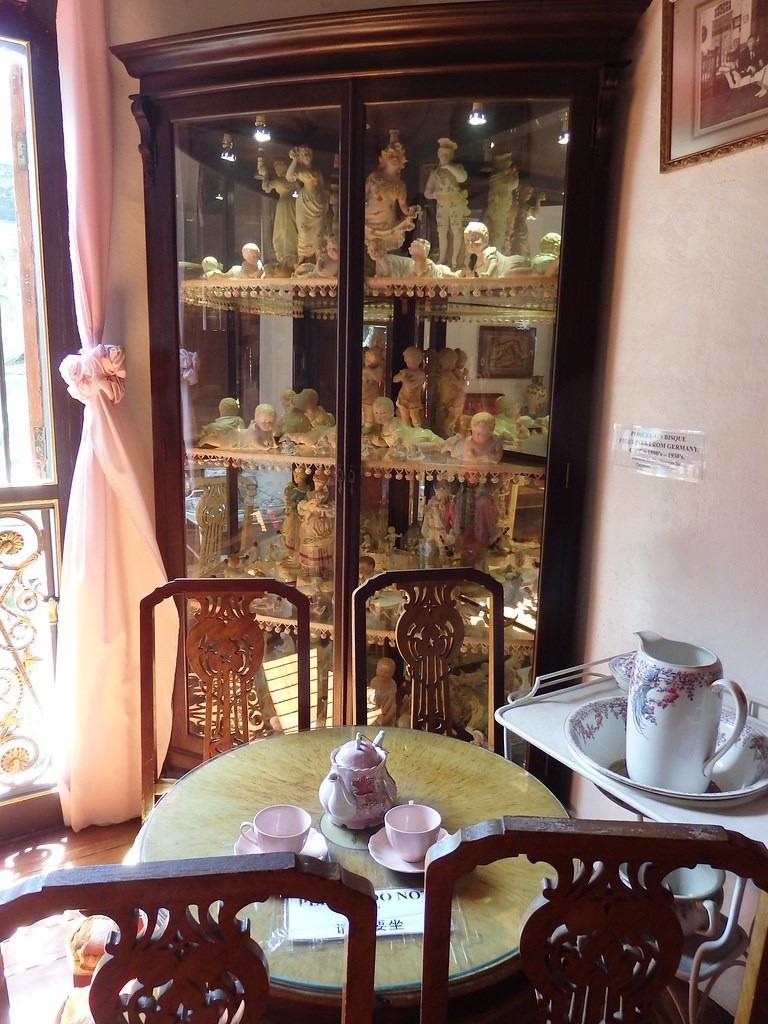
[625,630,747,793]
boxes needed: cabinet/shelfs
[491,652,768,1023]
[108,0,649,812]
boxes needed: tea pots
[319,730,397,829]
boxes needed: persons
[178,112,560,282]
[448,345,550,454]
[466,133,565,282]
[450,650,534,749]
[193,322,507,469]
[460,474,544,624]
[362,650,485,751]
[185,467,537,626]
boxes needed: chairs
[0,852,379,1022]
[137,578,311,827]
[351,568,505,760]
[418,814,768,1023]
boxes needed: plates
[368,826,451,873]
[564,695,768,808]
[234,827,329,861]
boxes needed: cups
[384,800,442,862]
[240,804,311,855]
[621,864,726,937]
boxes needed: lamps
[219,101,570,163]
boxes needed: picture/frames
[477,326,537,379]
[657,0,767,175]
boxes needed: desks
[122,724,580,1022]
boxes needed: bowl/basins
[608,651,638,692]
